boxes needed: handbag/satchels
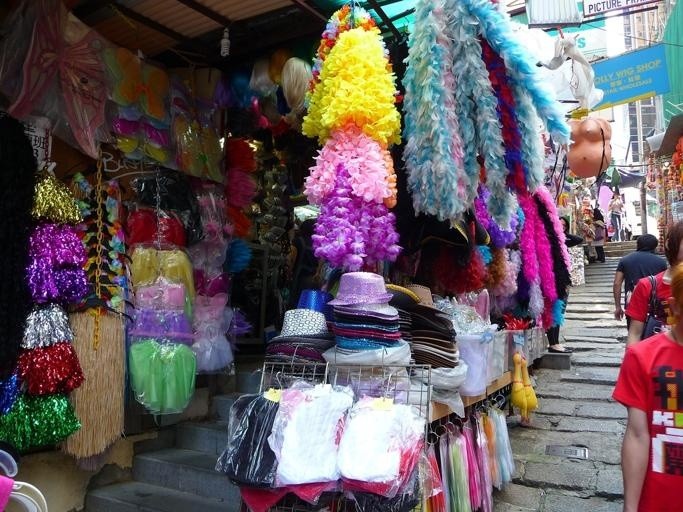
[641,275,661,340]
[592,225,602,241]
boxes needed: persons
[620,217,683,351]
[607,190,624,243]
[587,208,608,265]
[609,261,683,512]
[544,215,580,355]
[611,232,666,330]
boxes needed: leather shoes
[547,347,571,353]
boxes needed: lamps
[219,29,232,57]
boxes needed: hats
[264,271,459,389]
